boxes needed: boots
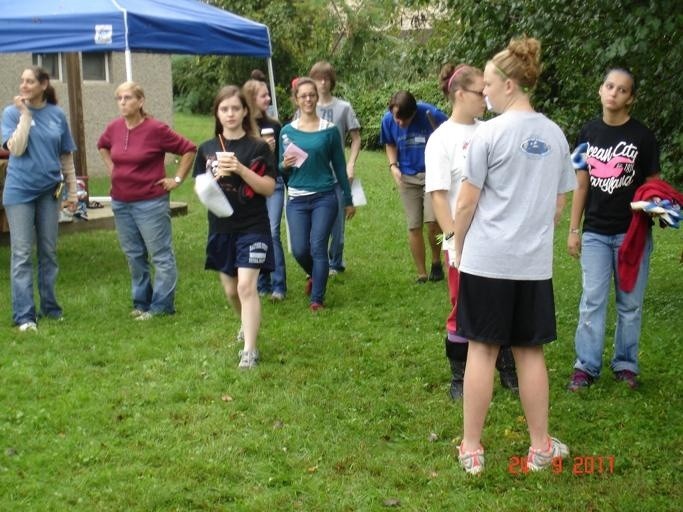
[445,338,468,402]
[496,344,519,393]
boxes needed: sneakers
[19,321,38,333]
[526,435,572,473]
[305,277,312,296]
[414,259,445,283]
[268,292,286,303]
[567,368,596,393]
[455,439,486,478]
[309,299,324,310]
[237,322,261,370]
[129,309,154,322]
[615,370,636,388]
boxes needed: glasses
[113,94,140,101]
[464,88,483,98]
[295,91,316,100]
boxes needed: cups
[214,151,236,175]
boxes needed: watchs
[389,162,400,168]
[172,175,183,184]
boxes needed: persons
[98,81,198,320]
[567,65,661,389]
[454,37,570,473]
[381,91,450,285]
[0,66,79,331]
[425,62,519,404]
[196,61,363,370]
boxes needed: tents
[0,0,292,253]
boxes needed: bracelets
[569,229,580,235]
[446,233,454,239]
